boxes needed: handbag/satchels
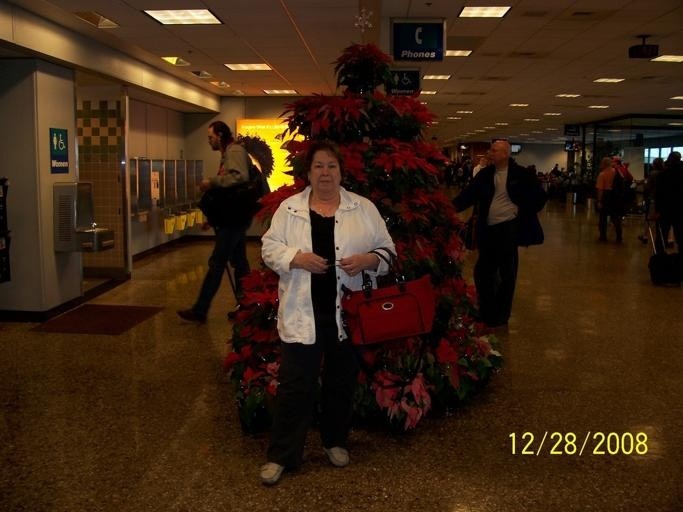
[197,166,269,232]
[612,172,625,193]
[340,273,437,345]
[461,214,478,251]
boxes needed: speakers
[636,133,644,147]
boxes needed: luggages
[648,221,679,287]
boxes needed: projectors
[628,44,659,60]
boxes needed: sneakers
[599,235,648,244]
[476,313,508,328]
[323,446,349,467]
[178,310,207,323]
[260,462,284,485]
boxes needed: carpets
[28,303,165,337]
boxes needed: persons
[638,157,674,248]
[652,151,683,254]
[446,156,488,191]
[593,155,634,243]
[176,120,253,326]
[260,138,397,485]
[552,164,565,176]
[450,140,547,326]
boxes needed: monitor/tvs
[565,140,579,152]
[510,143,522,154]
[564,123,580,136]
